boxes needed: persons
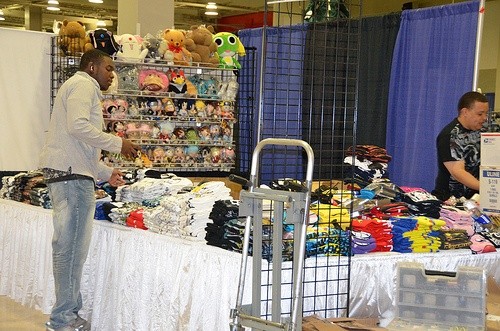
[432,91,490,203]
[46,49,142,331]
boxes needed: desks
[0,197,500,331]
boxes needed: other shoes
[47,317,91,331]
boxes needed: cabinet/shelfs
[49,34,240,176]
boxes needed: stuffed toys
[50,19,247,171]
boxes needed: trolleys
[228,139,500,331]
